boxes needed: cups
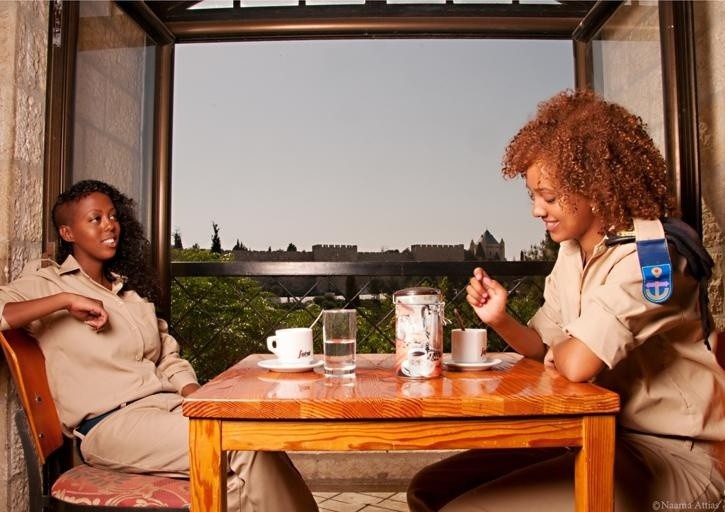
[266,327,314,365]
[323,309,357,379]
[450,328,487,363]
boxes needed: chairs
[1,329,191,510]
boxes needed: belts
[75,402,130,465]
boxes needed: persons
[407,93,725,512]
[1,180,319,512]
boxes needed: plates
[258,358,325,372]
[444,359,505,370]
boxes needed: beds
[182,351,621,512]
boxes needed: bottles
[393,286,444,379]
